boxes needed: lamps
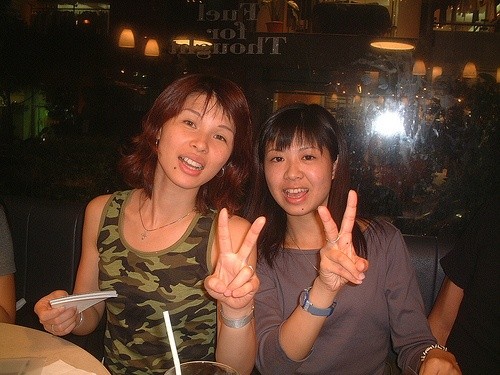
[370,41,415,50]
[145,40,159,56]
[175,39,212,47]
[413,60,426,75]
[432,67,442,80]
[118,30,134,48]
[463,63,477,78]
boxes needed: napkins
[50,290,118,314]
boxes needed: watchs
[299,287,337,316]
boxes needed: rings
[248,265,254,273]
[326,235,340,243]
[51,325,55,333]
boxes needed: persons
[253,102,462,375]
[33,74,266,375]
[0,204,17,323]
[426,192,500,375]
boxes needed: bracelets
[421,344,447,362]
[73,312,84,328]
[220,305,254,328]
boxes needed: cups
[164,361,239,375]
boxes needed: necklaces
[288,232,320,272]
[139,193,195,240]
